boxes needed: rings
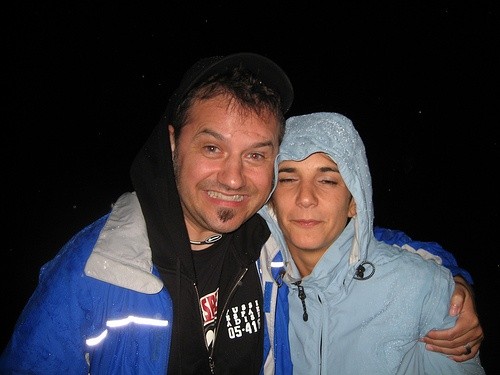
[464,344,471,355]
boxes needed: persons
[0,52,485,374]
[257,112,485,374]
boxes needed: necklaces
[190,233,222,245]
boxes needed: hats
[159,51,295,116]
